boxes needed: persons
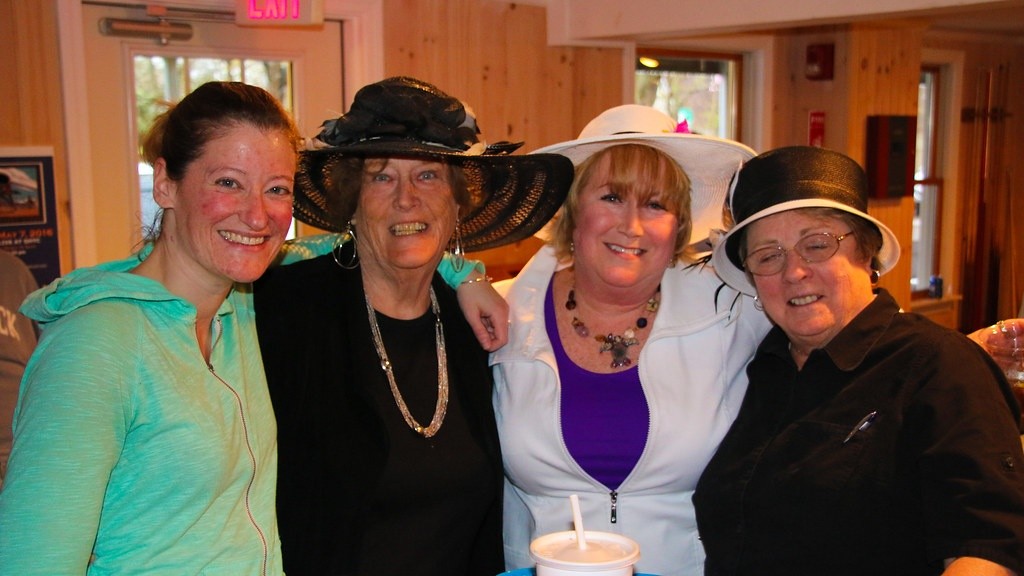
[488,105,773,576]
[0,81,513,575]
[692,146,1024,576]
[0,172,41,490]
[253,77,575,576]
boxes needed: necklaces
[565,264,661,369]
[364,285,449,437]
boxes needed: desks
[965,326,1024,434]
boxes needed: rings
[507,319,512,325]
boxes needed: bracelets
[458,275,493,284]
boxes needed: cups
[530,530,640,576]
[978,323,1024,435]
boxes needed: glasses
[742,231,853,276]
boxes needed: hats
[290,76,575,252]
[712,144,901,297]
[525,102,758,245]
